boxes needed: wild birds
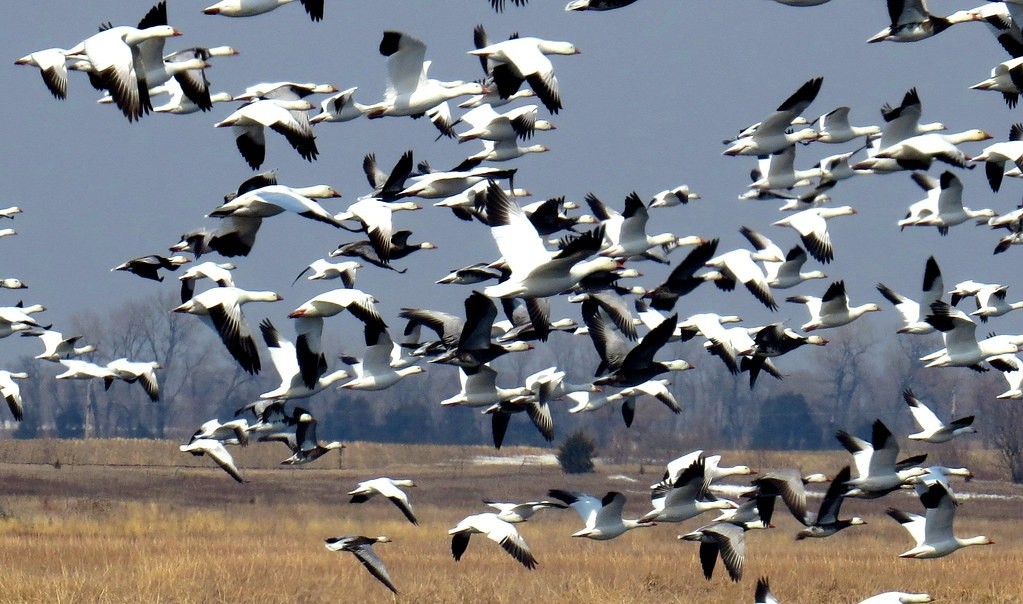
[14,0,637,158]
[0,207,162,420]
[723,0,1022,194]
[108,148,1023,603]
